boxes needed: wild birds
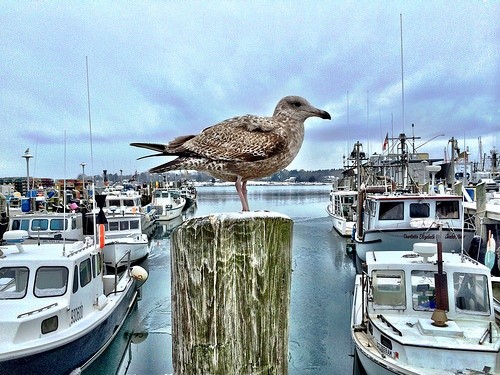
[129,95,332,212]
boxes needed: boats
[4,213,94,242]
[0,231,148,375]
[353,181,473,267]
[462,137,500,326]
[350,222,500,375]
[324,146,416,236]
[100,206,149,268]
[95,167,172,204]
[154,189,186,220]
[92,189,157,236]
[180,179,197,209]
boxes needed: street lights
[79,162,87,189]
[22,148,35,191]
[119,168,123,181]
